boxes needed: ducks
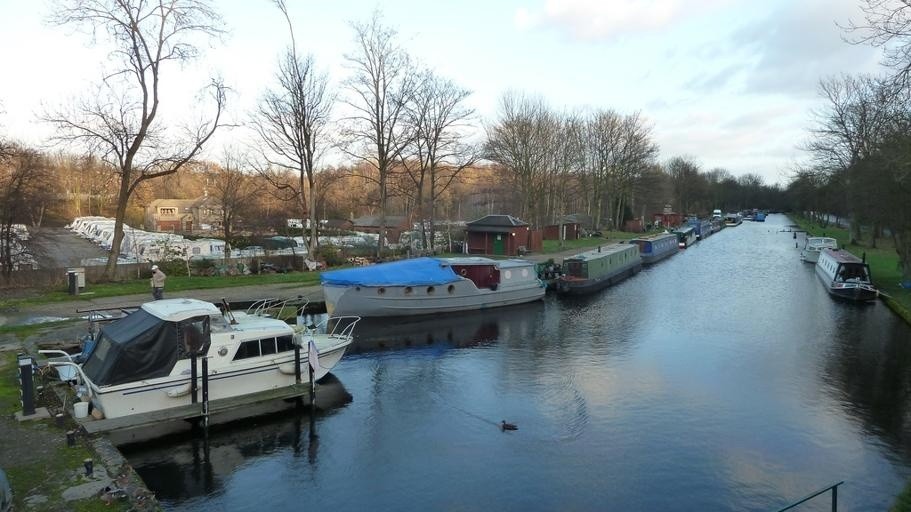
[502,420,519,431]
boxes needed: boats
[553,239,645,297]
[314,251,551,322]
[799,234,838,265]
[630,207,769,266]
[32,292,370,452]
[115,371,356,504]
[323,297,550,359]
[812,242,879,302]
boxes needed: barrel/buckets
[73,401,89,419]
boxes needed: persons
[150,265,168,300]
[536,262,565,281]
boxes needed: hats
[151,265,158,270]
